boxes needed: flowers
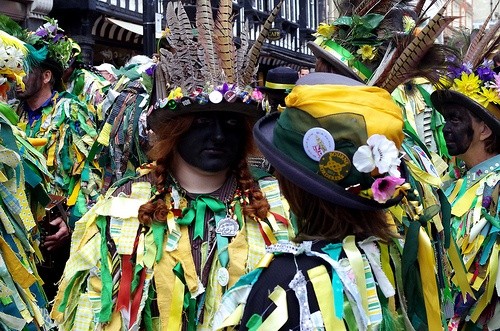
[352,134,411,206]
[0,14,500,112]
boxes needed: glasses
[94,71,109,75]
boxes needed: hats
[252,0,465,216]
[258,67,299,94]
[308,0,452,83]
[429,0,500,132]
[0,11,48,96]
[23,16,81,79]
[147,1,285,135]
[93,63,118,80]
[125,55,152,69]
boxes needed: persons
[202,77,444,331]
[256,67,299,115]
[46,43,285,331]
[14,16,105,331]
[0,13,56,331]
[431,58,500,331]
[93,63,117,85]
[314,23,387,86]
[152,52,160,65]
[298,66,310,79]
[124,55,153,78]
